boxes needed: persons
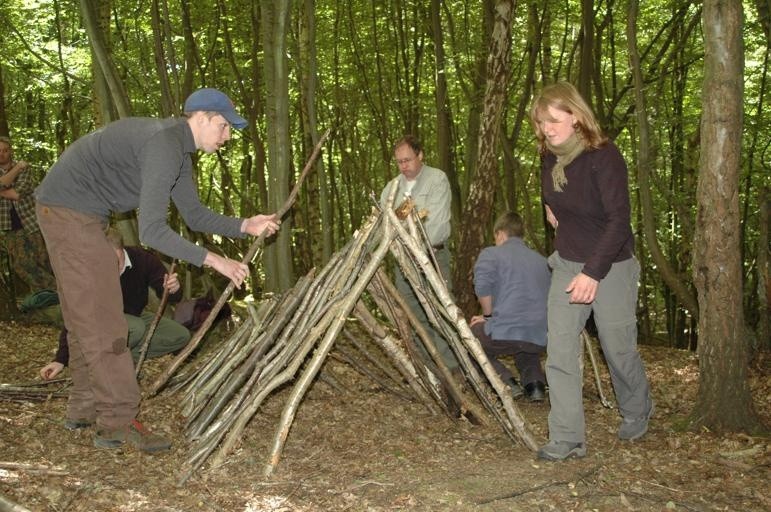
[469,212,552,401]
[529,84,654,461]
[33,87,282,452]
[0,135,56,292]
[39,226,190,384]
[381,136,459,373]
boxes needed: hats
[183,88,249,130]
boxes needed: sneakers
[537,440,587,462]
[618,399,656,441]
[65,418,96,430]
[93,417,172,452]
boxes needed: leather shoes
[525,382,545,402]
[505,378,525,400]
